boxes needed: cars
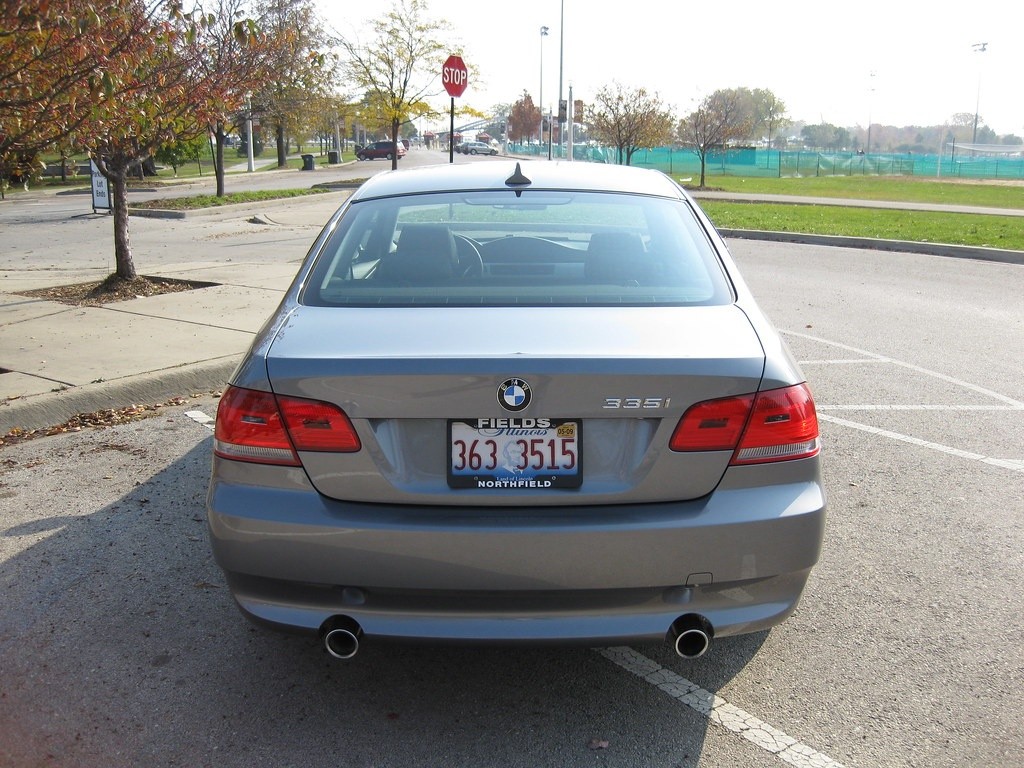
[356,139,409,160]
[456,142,499,156]
[204,160,827,660]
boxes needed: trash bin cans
[355,145,362,155]
[328,151,339,164]
[301,155,313,170]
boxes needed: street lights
[243,90,254,171]
[567,79,574,159]
[537,25,549,148]
[332,105,343,162]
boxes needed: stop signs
[442,55,468,97]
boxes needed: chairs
[392,224,463,284]
[583,231,649,284]
